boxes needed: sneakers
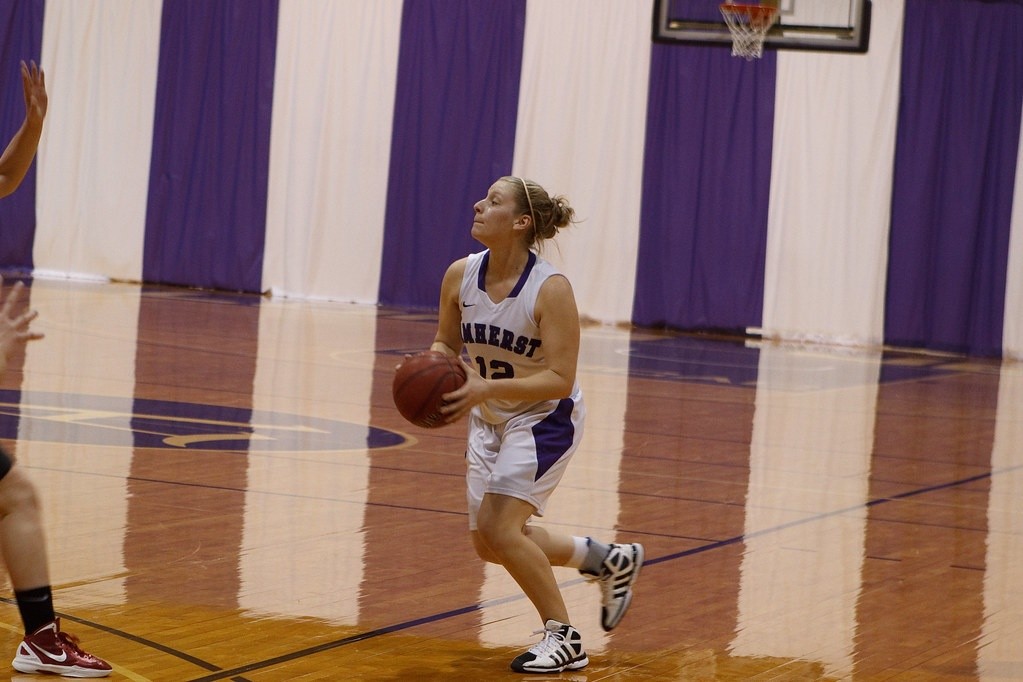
[509,618,588,673]
[579,542,644,632]
[11,616,113,678]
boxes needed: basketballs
[394,348,468,428]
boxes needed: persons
[394,175,645,675]
[0,58,113,678]
